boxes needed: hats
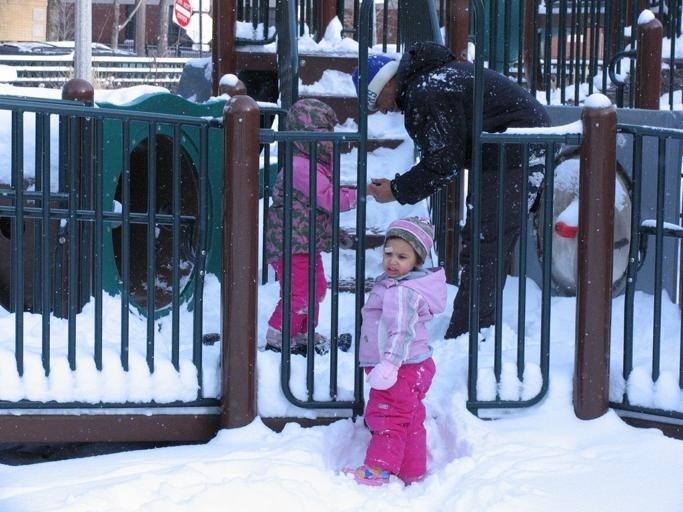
[352,54,399,111]
[384,216,435,264]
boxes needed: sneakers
[340,465,390,486]
[265,332,330,358]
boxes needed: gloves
[366,359,399,391]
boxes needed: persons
[347,216,447,486]
[266,100,357,356]
[353,39,553,338]
